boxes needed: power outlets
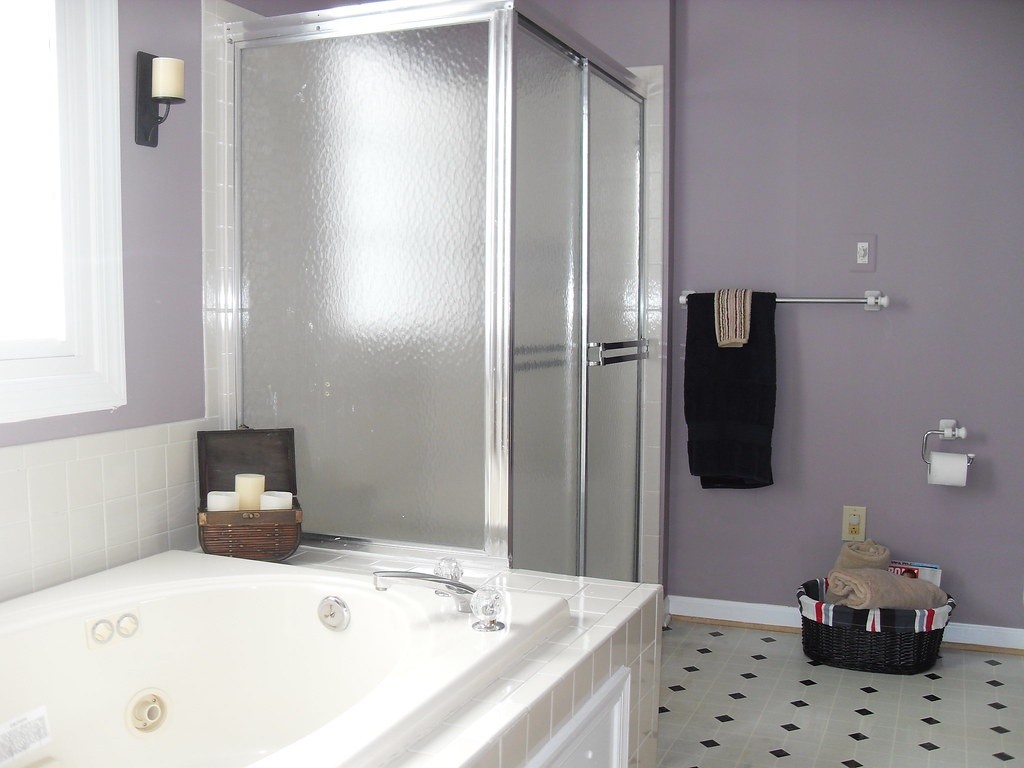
[841,506,867,544]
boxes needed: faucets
[371,569,476,604]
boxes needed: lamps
[133,51,186,148]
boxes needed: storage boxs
[196,428,302,564]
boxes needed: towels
[827,568,947,609]
[235,473,265,510]
[207,490,239,511]
[712,289,752,349]
[684,292,777,489]
[260,492,292,509]
[822,542,891,605]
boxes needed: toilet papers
[928,452,967,486]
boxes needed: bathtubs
[2,526,664,767]
